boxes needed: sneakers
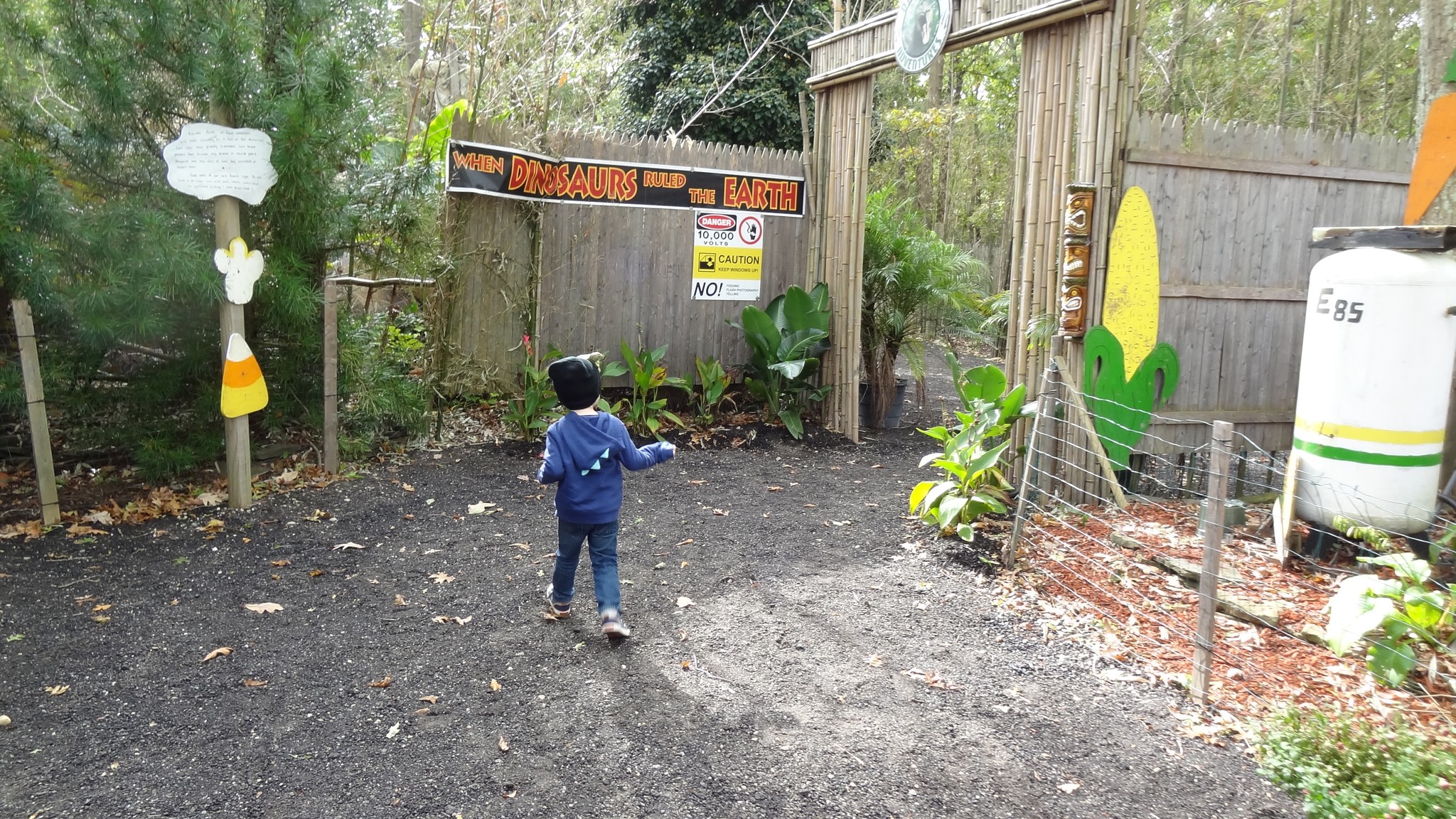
[547,583,571,615]
[599,608,630,640]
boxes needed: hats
[547,355,601,410]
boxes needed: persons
[537,357,676,637]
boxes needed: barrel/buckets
[1195,500,1246,543]
